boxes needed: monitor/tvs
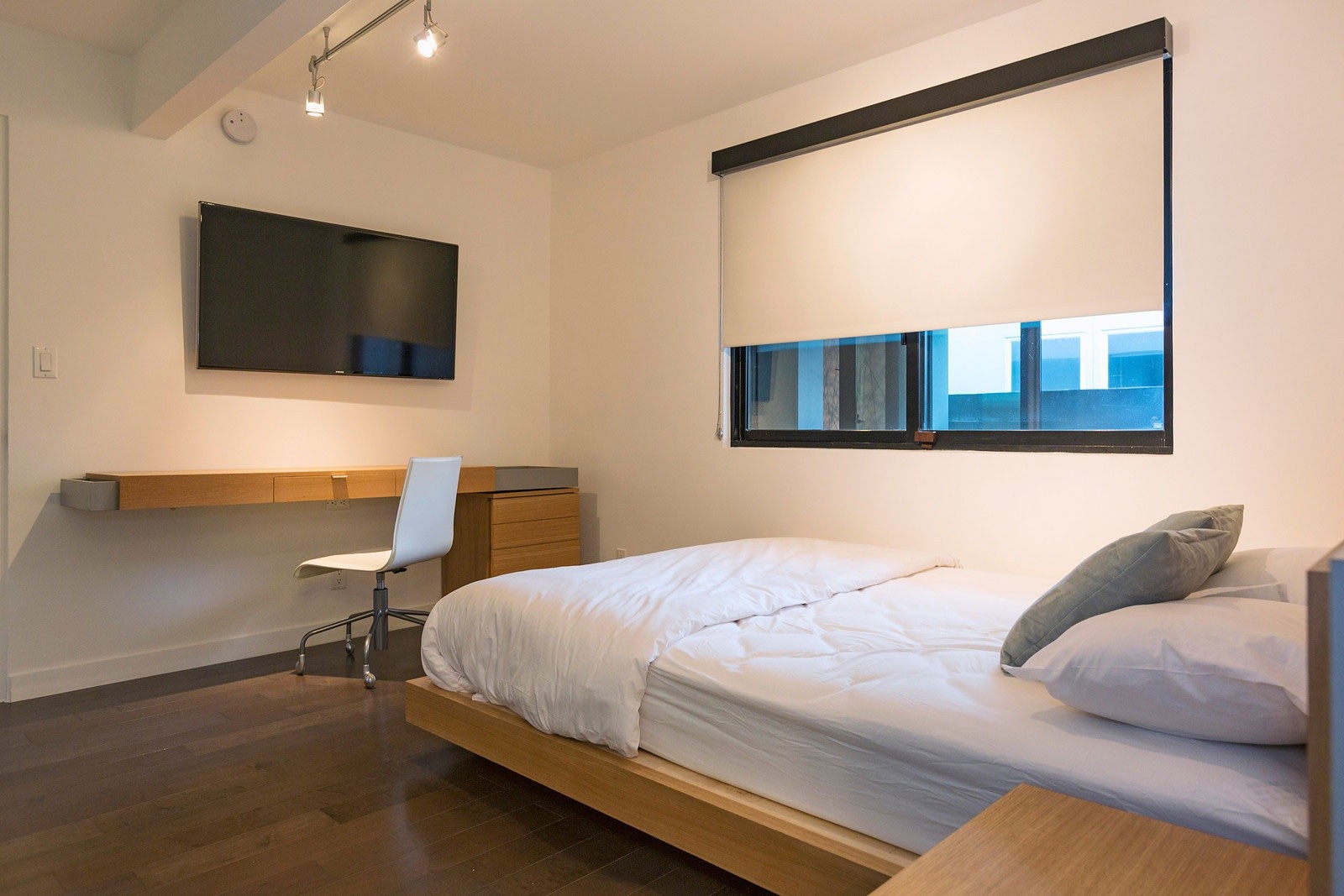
[197,200,459,381]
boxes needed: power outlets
[326,500,350,511]
[330,570,348,590]
[616,548,627,559]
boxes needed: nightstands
[872,782,1309,896]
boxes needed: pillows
[995,503,1325,744]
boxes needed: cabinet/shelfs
[441,488,582,600]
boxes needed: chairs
[293,456,464,688]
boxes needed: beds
[403,538,1344,896]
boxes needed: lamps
[305,54,326,117]
[413,0,448,57]
